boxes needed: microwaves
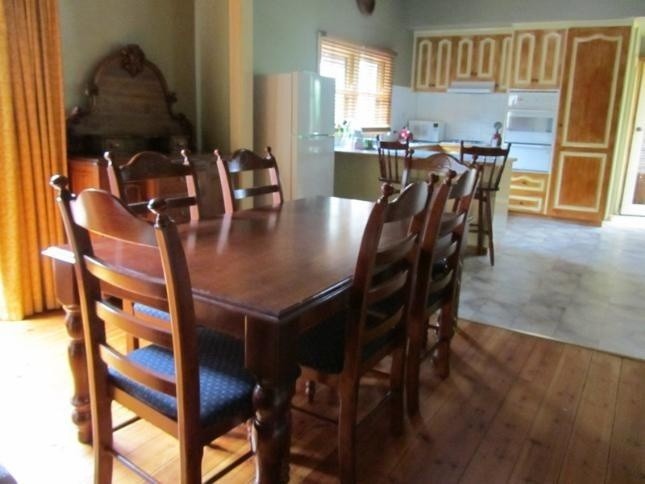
[408,119,445,142]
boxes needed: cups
[365,139,375,149]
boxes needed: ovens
[500,92,560,172]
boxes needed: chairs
[373,163,483,412]
[375,134,413,195]
[450,140,511,266]
[289,171,441,484]
[213,146,284,212]
[103,148,205,356]
[51,173,301,484]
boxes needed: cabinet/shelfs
[412,28,514,92]
[507,172,549,214]
[69,152,228,309]
[512,28,565,90]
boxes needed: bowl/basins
[378,133,400,142]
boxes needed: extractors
[447,81,496,94]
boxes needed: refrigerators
[255,72,336,206]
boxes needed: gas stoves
[438,140,483,145]
